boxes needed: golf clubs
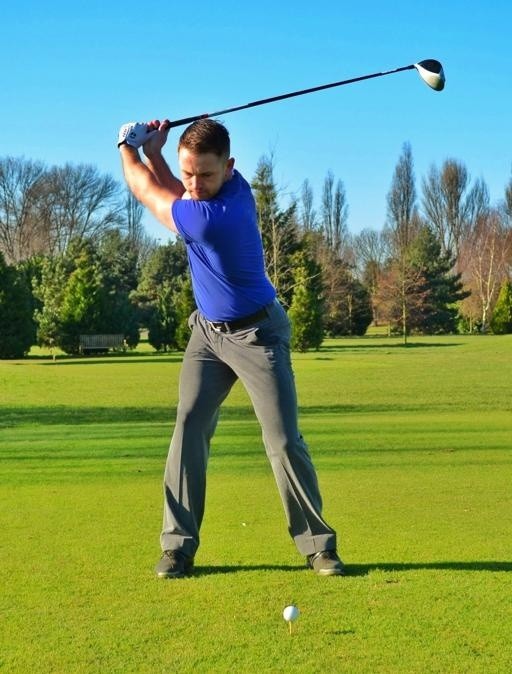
[168,59,445,128]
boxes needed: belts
[203,298,280,335]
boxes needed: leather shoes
[307,548,344,577]
[157,550,195,579]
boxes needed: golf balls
[283,606,299,620]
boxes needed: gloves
[116,120,158,147]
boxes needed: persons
[116,113,344,584]
